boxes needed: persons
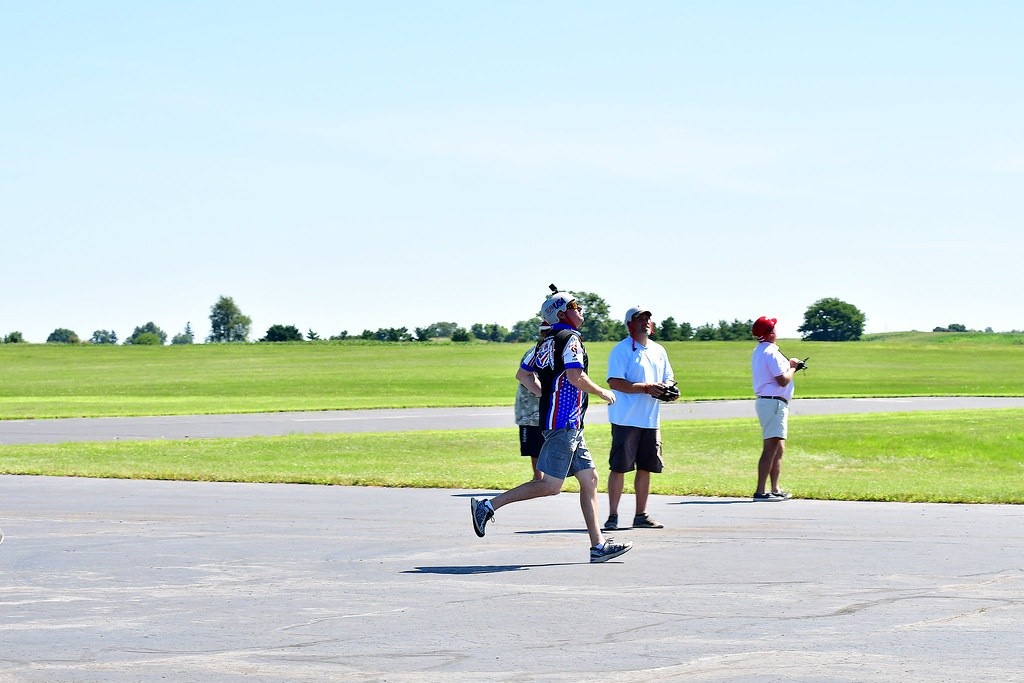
[750,316,801,502]
[470,293,633,563]
[605,308,681,530]
[514,320,553,482]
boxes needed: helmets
[541,291,576,325]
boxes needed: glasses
[566,306,582,312]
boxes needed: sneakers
[590,537,634,563]
[753,492,784,501]
[605,514,619,529]
[772,491,792,499]
[632,512,664,528]
[470,496,494,537]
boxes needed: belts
[761,395,789,405]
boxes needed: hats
[539,325,551,335]
[752,316,777,342]
[625,305,652,325]
